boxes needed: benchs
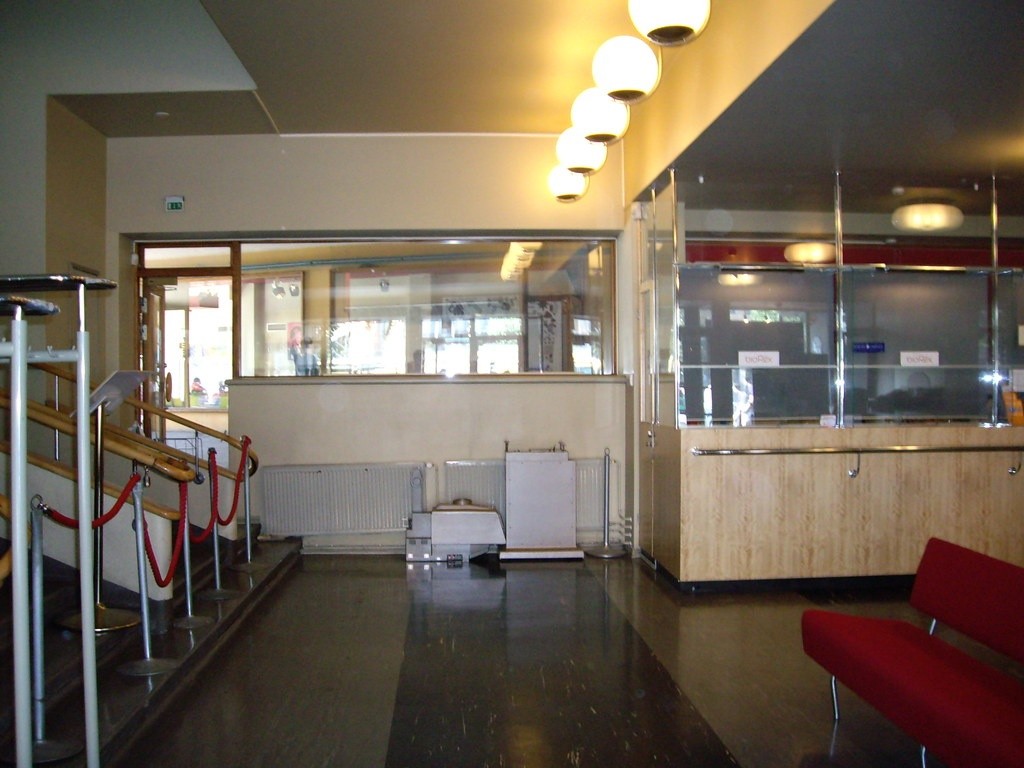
[805,536,1024,768]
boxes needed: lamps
[891,197,964,233]
[557,125,607,175]
[572,88,630,144]
[591,35,662,103]
[628,0,711,46]
[549,165,590,203]
[500,241,543,281]
[784,242,835,263]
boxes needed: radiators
[445,458,632,546]
[263,462,440,536]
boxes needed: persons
[413,349,425,373]
[290,336,321,376]
